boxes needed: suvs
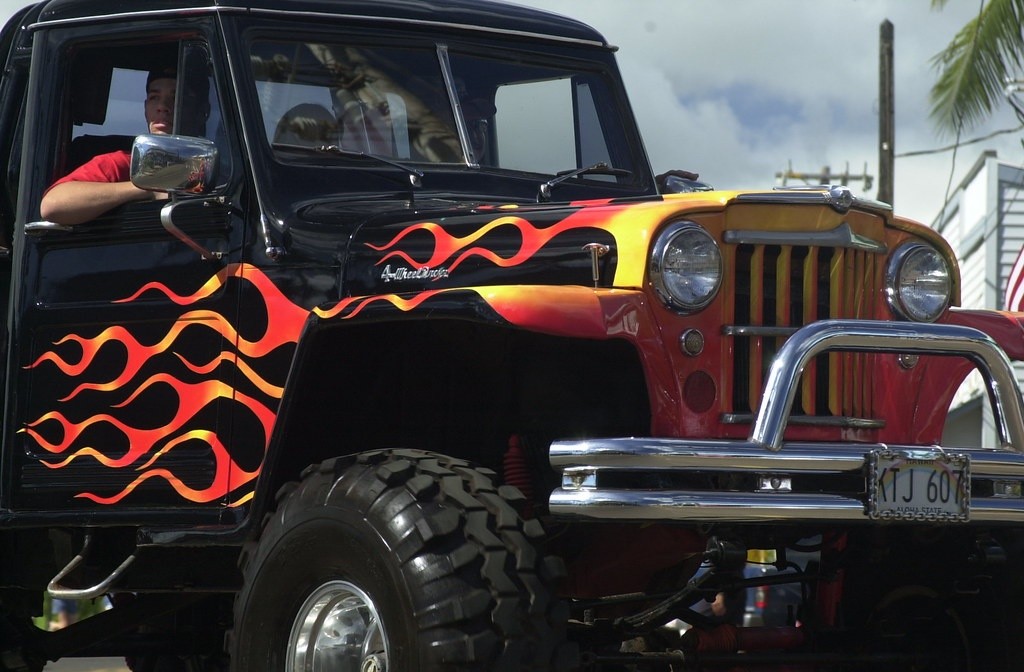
[0,0,1024,672]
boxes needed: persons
[40,54,211,225]
[700,592,742,627]
[457,91,497,162]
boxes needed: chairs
[66,135,141,172]
